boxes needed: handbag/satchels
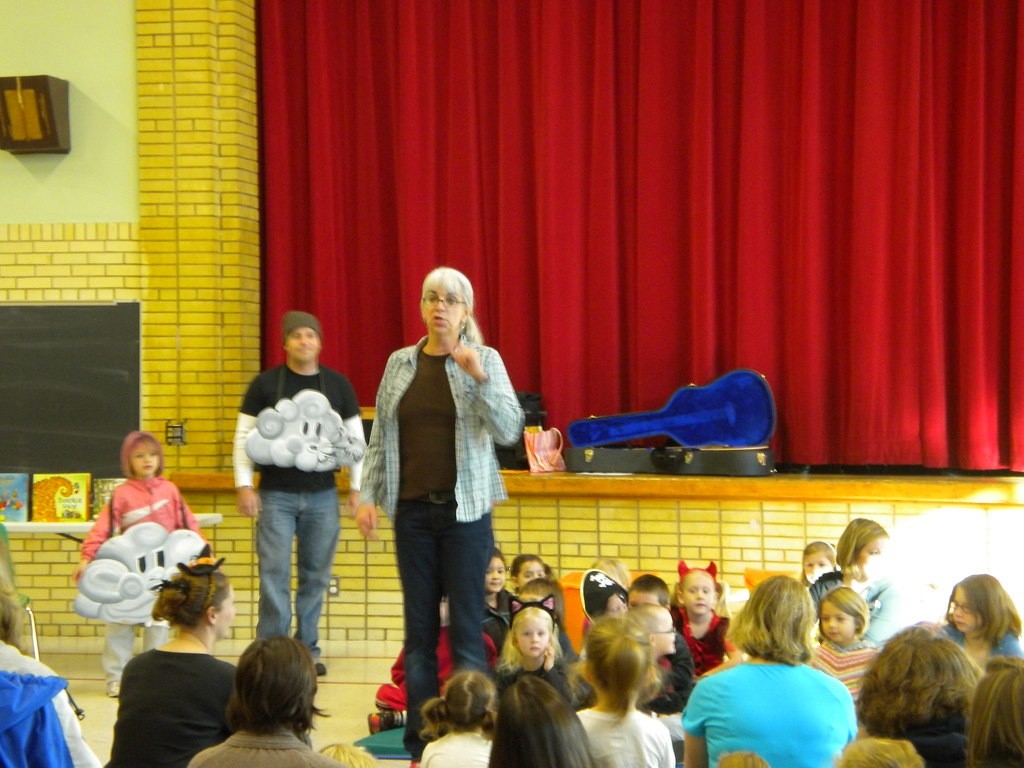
[523,428,566,473]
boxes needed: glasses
[655,629,676,635]
[949,601,974,614]
[421,295,467,308]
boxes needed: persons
[860,624,986,768]
[357,266,525,768]
[800,541,840,587]
[232,311,367,674]
[188,634,374,768]
[71,430,209,697]
[418,670,499,768]
[683,574,858,768]
[0,538,103,768]
[488,676,592,768]
[942,574,1024,663]
[809,517,898,649]
[811,587,876,703]
[837,738,925,768]
[105,544,237,768]
[670,560,742,677]
[575,617,676,768]
[367,548,572,732]
[967,655,1024,768]
[579,558,694,715]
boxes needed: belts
[417,490,454,505]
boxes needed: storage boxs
[32,472,91,522]
[0,472,30,522]
[92,478,128,520]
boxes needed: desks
[0,513,224,545]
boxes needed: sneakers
[369,710,402,734]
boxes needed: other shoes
[314,663,325,675]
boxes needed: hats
[280,311,322,343]
[581,569,629,625]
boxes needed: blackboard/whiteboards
[0,299,140,479]
